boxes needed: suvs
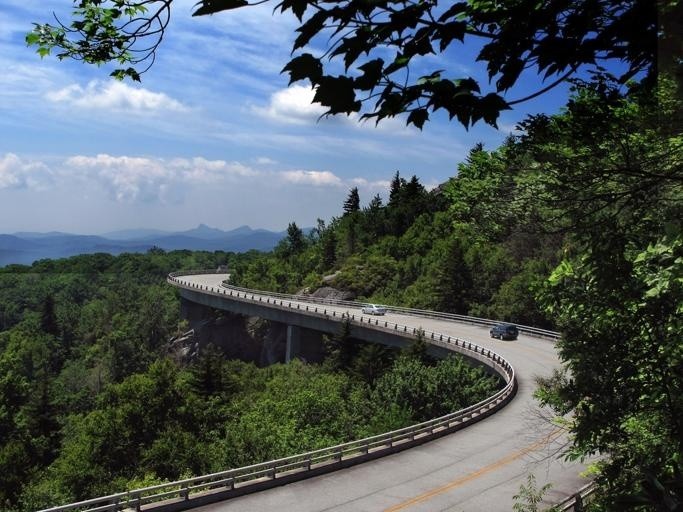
[489,324,519,340]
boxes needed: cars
[360,304,387,316]
